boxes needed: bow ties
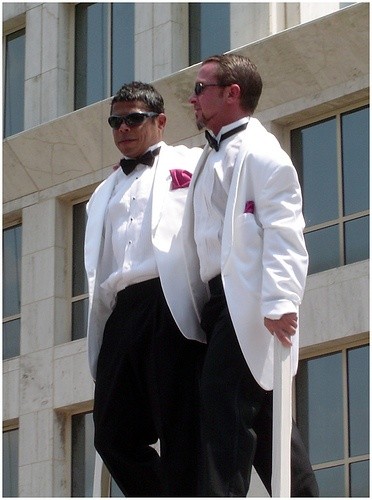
[203,131,223,153]
[120,148,161,175]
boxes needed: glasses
[194,81,220,93]
[108,110,159,127]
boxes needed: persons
[85,81,203,498]
[182,53,318,498]
